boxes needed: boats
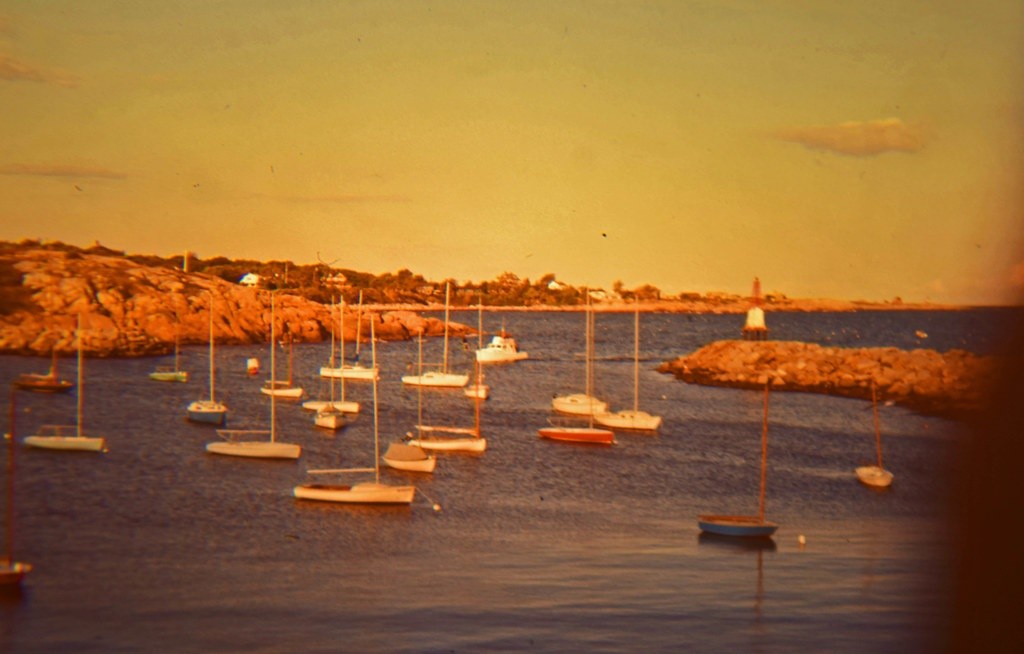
[471,318,529,363]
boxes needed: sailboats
[1,391,33,587]
[693,383,782,542]
[206,294,302,459]
[533,286,665,445]
[21,313,108,452]
[183,295,233,425]
[17,343,73,393]
[408,295,491,453]
[291,312,422,505]
[381,326,440,477]
[256,280,385,428]
[463,293,493,401]
[854,371,897,487]
[400,280,474,389]
[147,295,191,383]
